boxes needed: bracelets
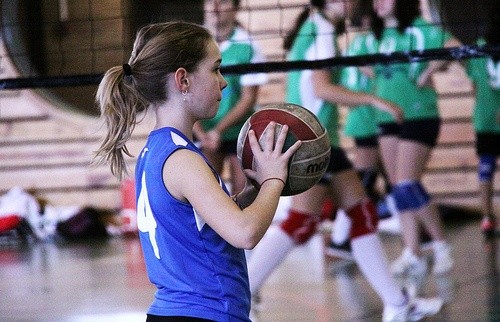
[259,177,287,190]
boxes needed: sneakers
[382,283,444,322]
[248,291,263,322]
[432,245,455,277]
[388,248,428,278]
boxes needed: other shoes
[325,237,355,263]
[314,218,334,233]
[480,214,496,234]
[375,217,401,236]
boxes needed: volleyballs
[236,102,330,196]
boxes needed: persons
[190,0,273,201]
[451,36,500,237]
[92,20,302,322]
[240,0,445,322]
[339,0,462,277]
